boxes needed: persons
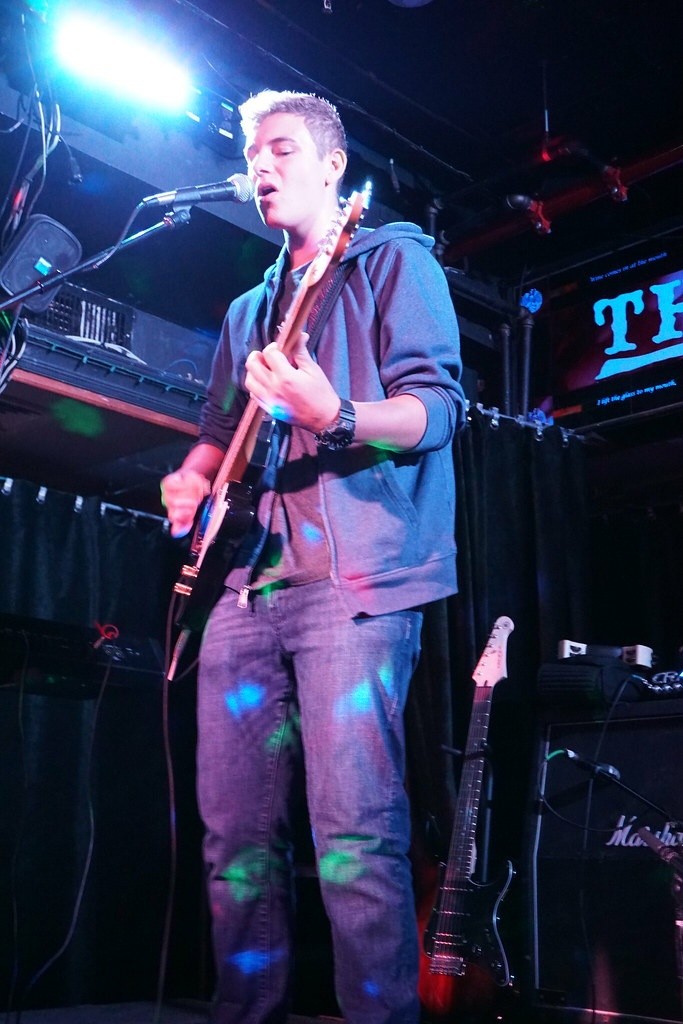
[161,90,464,1024]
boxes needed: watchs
[314,398,357,453]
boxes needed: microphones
[143,173,256,208]
[564,748,621,781]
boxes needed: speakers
[0,213,83,314]
[527,698,683,1024]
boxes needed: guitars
[169,177,376,627]
[411,614,524,1024]
